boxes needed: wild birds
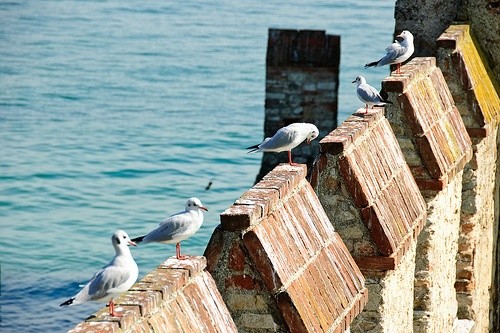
[130,196,209,260]
[352,75,394,115]
[246,122,320,166]
[364,29,414,75]
[59,230,139,317]
